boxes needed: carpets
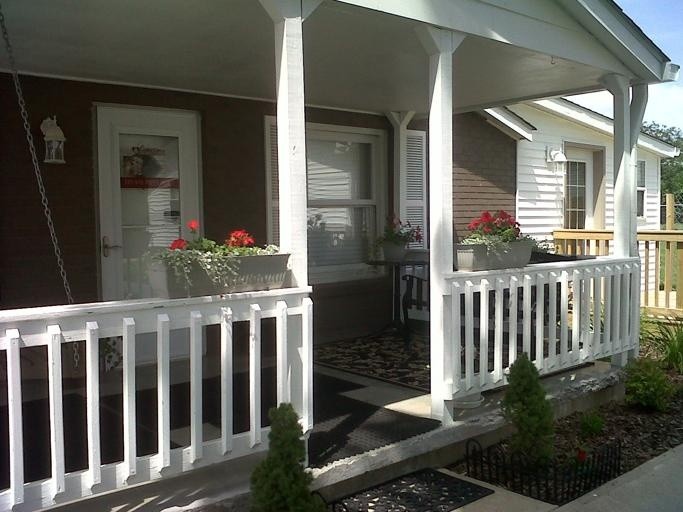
[327,467,495,512]
[312,323,561,395]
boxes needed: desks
[365,260,429,337]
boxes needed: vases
[383,240,405,261]
[163,247,291,298]
[456,241,538,272]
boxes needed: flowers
[374,213,424,252]
[150,220,279,288]
[460,209,536,256]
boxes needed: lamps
[39,115,67,165]
[546,143,568,165]
